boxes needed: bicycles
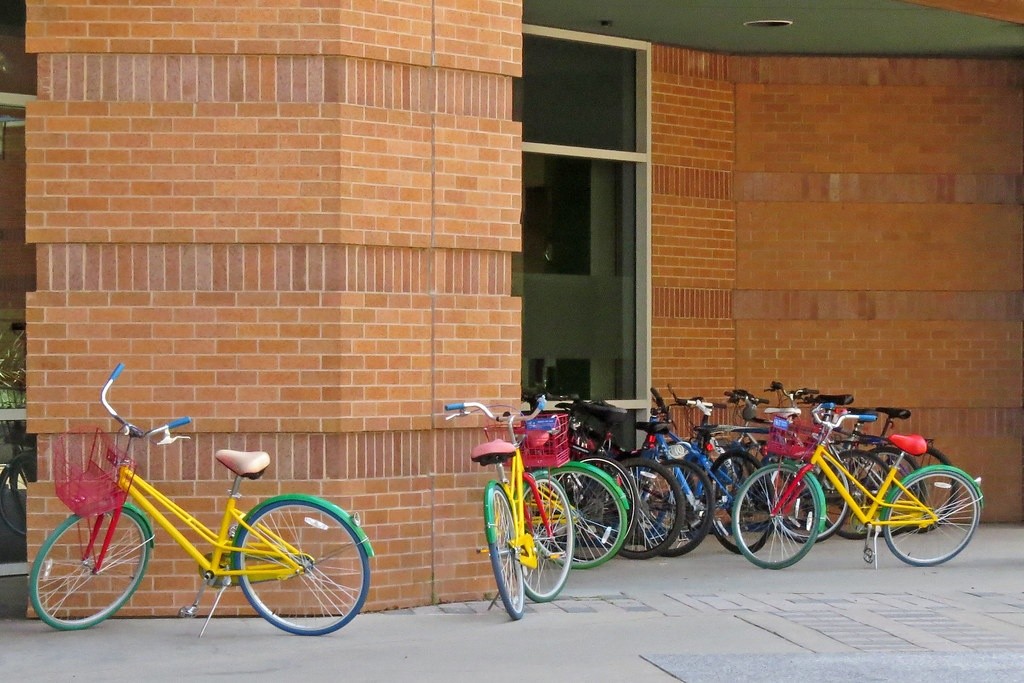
[521,378,986,573]
[26,361,376,638]
[0,321,37,538]
[444,391,576,622]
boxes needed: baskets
[766,415,820,460]
[53,424,138,520]
[483,414,570,468]
